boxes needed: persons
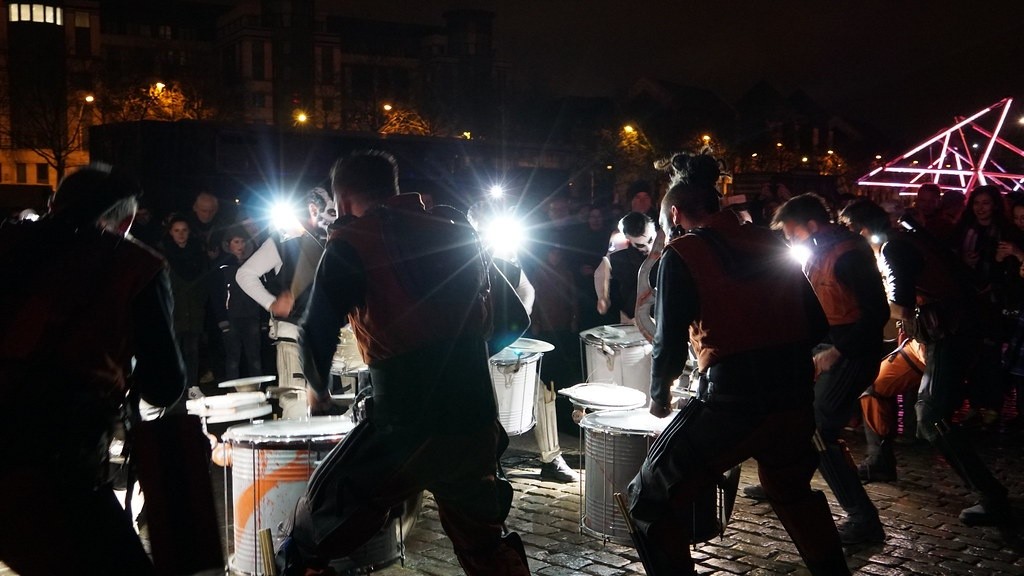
[625,179,855,576]
[0,164,188,576]
[743,191,885,542]
[838,197,1012,525]
[271,149,534,576]
[140,181,1023,486]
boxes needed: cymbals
[507,338,555,353]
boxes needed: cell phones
[206,241,216,252]
[999,226,1009,242]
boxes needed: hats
[627,180,652,201]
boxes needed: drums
[579,323,652,409]
[557,383,723,545]
[184,391,409,575]
[488,338,555,436]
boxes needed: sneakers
[744,484,767,498]
[838,516,887,544]
[540,458,580,482]
[960,500,1011,524]
[857,457,897,481]
